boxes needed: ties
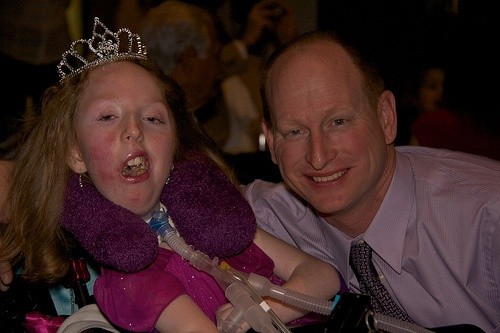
[349,241,421,329]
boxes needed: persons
[1,18,343,333]
[410,1,500,164]
[10,27,500,332]
[2,0,80,151]
[403,61,447,148]
[217,0,298,186]
[131,1,243,195]
